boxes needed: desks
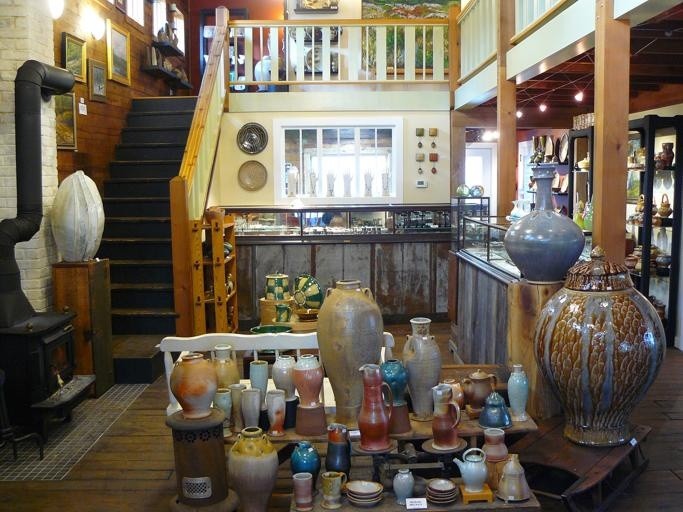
[163,365,546,512]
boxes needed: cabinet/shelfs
[143,47,191,89]
[448,186,493,324]
[191,218,238,337]
[157,27,184,58]
[300,48,341,76]
[531,135,572,235]
[302,25,343,43]
[454,213,574,426]
[204,25,247,91]
[564,118,683,349]
[52,259,111,398]
[209,204,489,323]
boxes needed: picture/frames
[362,0,460,75]
[63,31,86,83]
[293,0,339,13]
[197,6,249,86]
[88,58,109,104]
[109,17,132,87]
[56,92,77,151]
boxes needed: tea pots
[427,383,462,452]
[358,363,395,452]
[635,195,644,213]
[463,368,497,414]
[453,447,489,495]
[658,192,672,219]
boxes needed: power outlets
[415,180,426,189]
[79,97,88,116]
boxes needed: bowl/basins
[294,309,318,323]
[292,274,322,313]
[246,324,293,352]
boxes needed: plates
[346,481,382,509]
[236,123,269,157]
[561,174,573,195]
[557,132,570,164]
[426,480,460,509]
[549,171,560,193]
[236,159,268,193]
[543,136,555,161]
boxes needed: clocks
[304,47,332,70]
